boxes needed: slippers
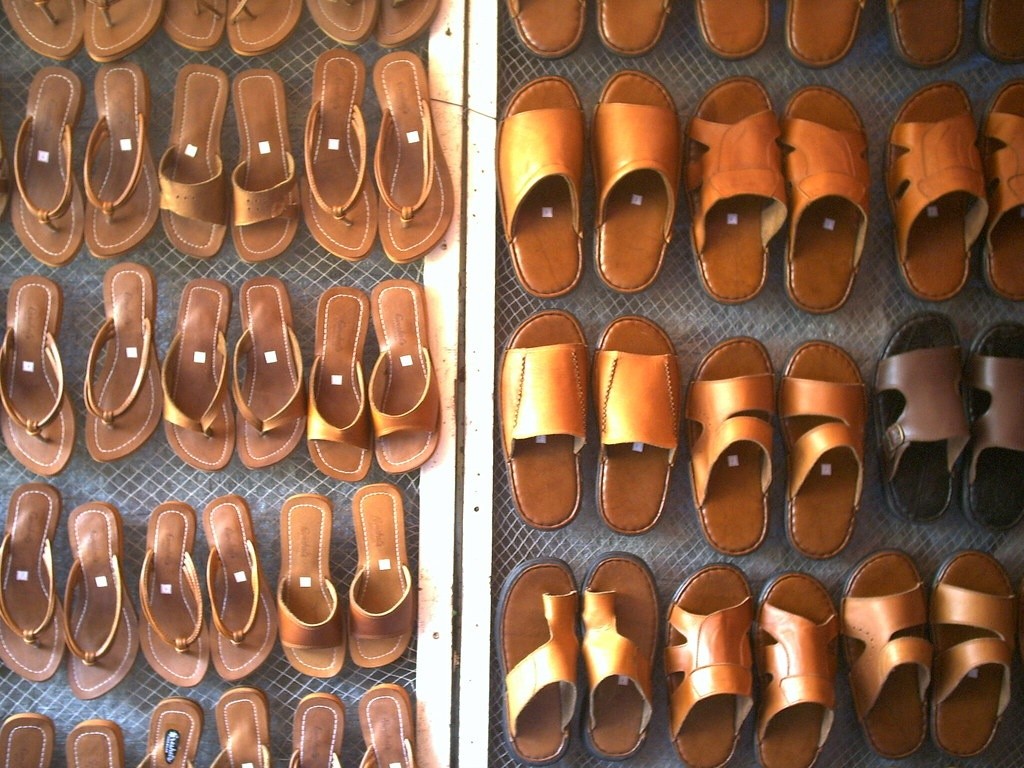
[0,0,1024,768]
